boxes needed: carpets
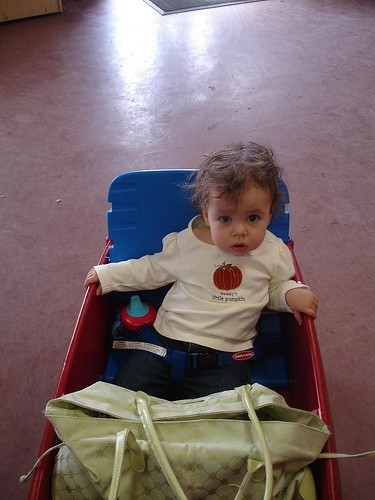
[143,0,258,16]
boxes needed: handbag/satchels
[22,380,329,500]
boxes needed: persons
[82,140,318,401]
